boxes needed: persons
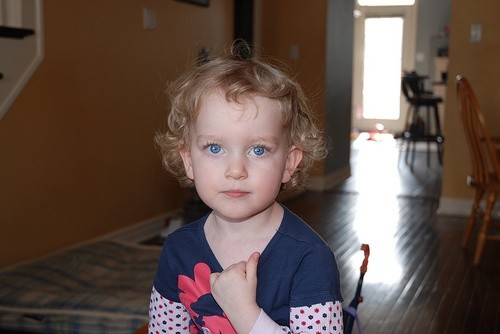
[150,56,344,334]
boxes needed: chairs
[451,74,500,266]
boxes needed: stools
[399,76,445,175]
[405,70,434,151]
